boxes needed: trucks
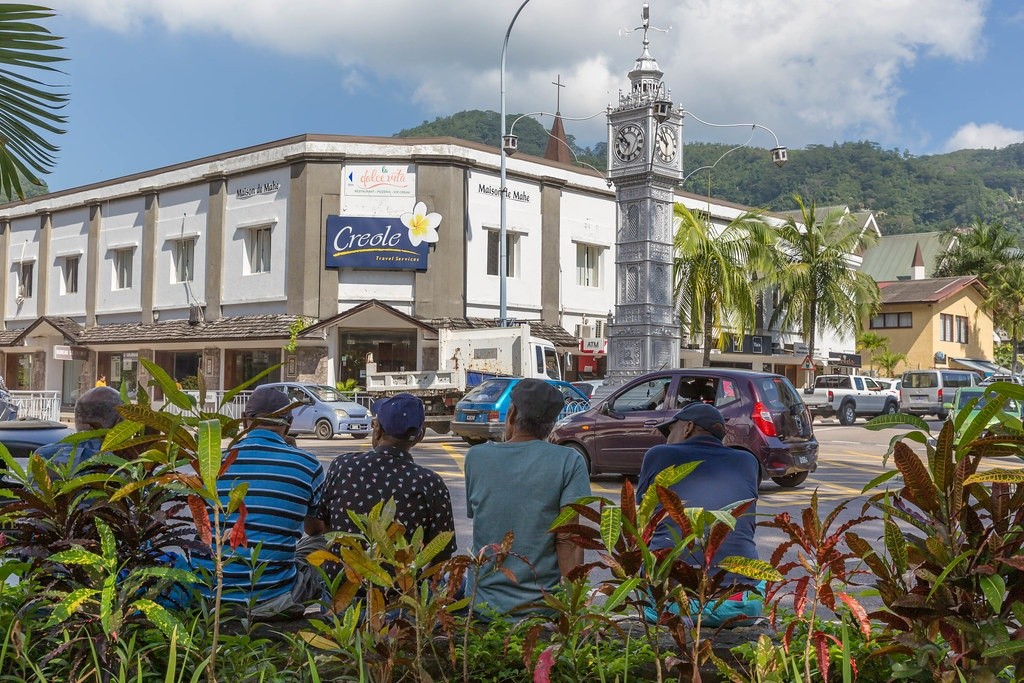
[979,374,1024,386]
[366,323,573,435]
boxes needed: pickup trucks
[794,374,900,426]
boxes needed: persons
[702,385,713,404]
[188,385,323,618]
[463,377,592,625]
[635,402,766,628]
[315,392,458,625]
[655,383,670,409]
[96,374,106,386]
[22,385,193,611]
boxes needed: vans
[900,369,985,421]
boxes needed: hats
[510,378,564,419]
[371,393,425,441]
[246,387,292,425]
[654,403,726,440]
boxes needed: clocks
[653,123,679,165]
[615,123,646,163]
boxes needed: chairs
[677,382,695,408]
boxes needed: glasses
[371,418,385,433]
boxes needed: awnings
[955,359,1019,377]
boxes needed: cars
[943,387,1024,453]
[449,377,592,446]
[561,379,606,402]
[547,367,819,488]
[865,378,907,393]
[255,381,373,440]
